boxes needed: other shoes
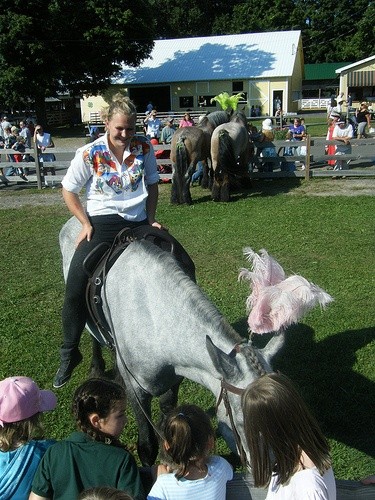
[52,185,56,189]
[45,181,48,186]
[327,166,333,170]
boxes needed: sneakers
[53,353,83,388]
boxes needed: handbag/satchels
[369,127,375,133]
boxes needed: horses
[171,109,234,206]
[59,212,286,469]
[210,106,249,202]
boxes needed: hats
[336,119,346,124]
[152,110,157,115]
[11,126,18,132]
[4,116,7,119]
[0,376,57,427]
[262,119,273,130]
[332,113,340,117]
[167,118,174,123]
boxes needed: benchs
[85,111,209,136]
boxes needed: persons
[51,91,198,388]
[28,377,145,500]
[275,96,281,110]
[241,372,337,500]
[179,113,195,129]
[284,116,308,171]
[188,111,212,188]
[146,405,233,500]
[90,124,100,143]
[255,118,297,179]
[0,375,57,500]
[326,92,352,122]
[324,115,354,180]
[0,113,57,190]
[242,105,262,117]
[160,117,176,175]
[141,109,164,144]
[357,101,371,140]
